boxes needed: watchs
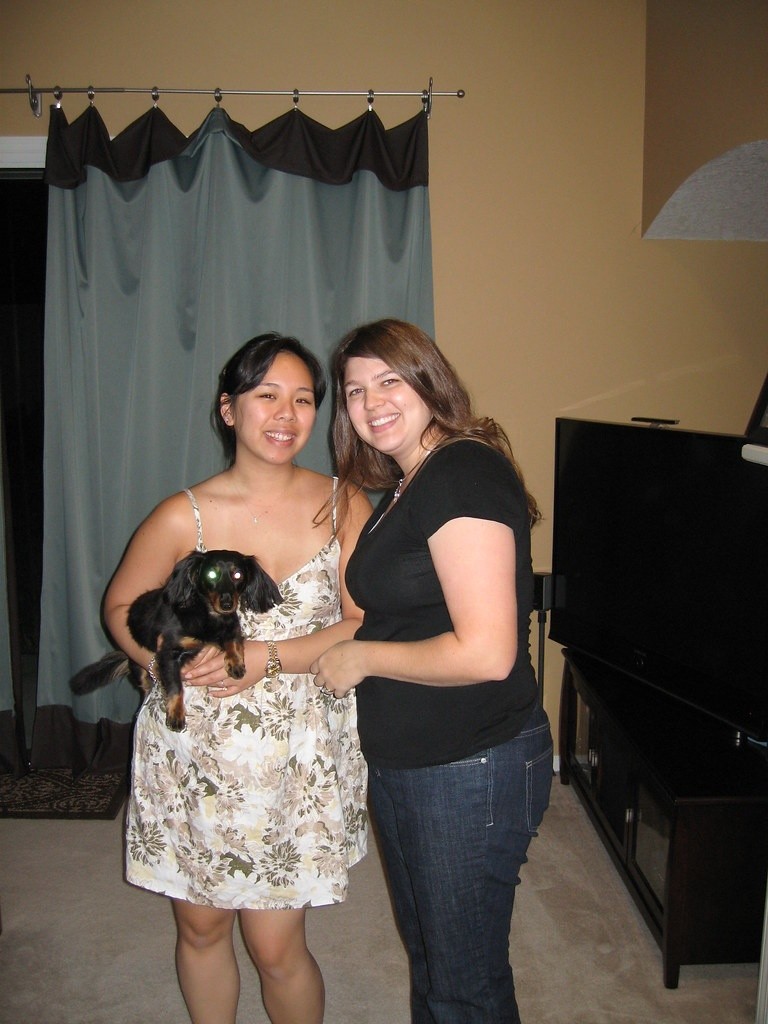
[264,640,282,678]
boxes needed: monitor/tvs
[549,418,768,749]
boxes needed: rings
[323,684,334,695]
[221,680,225,689]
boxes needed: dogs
[66,549,286,733]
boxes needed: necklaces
[394,432,446,503]
[230,466,297,523]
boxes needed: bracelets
[148,656,158,682]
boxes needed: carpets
[0,733,136,820]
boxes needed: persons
[101,329,374,1024]
[310,318,553,1024]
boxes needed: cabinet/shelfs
[559,649,768,991]
